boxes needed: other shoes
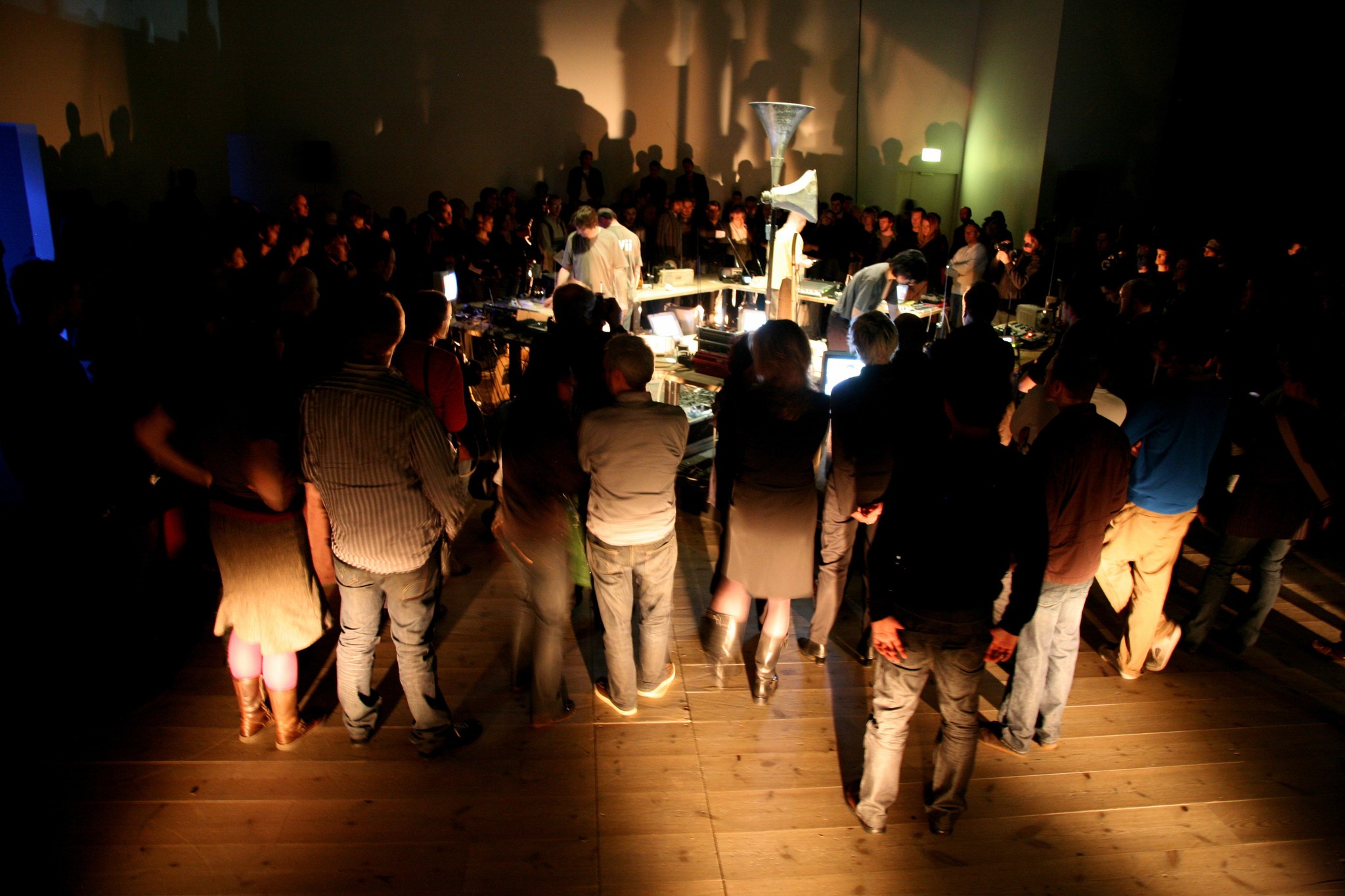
[1146,625,1180,672]
[845,781,886,834]
[922,780,952,834]
[421,718,482,764]
[595,676,637,716]
[638,660,674,696]
[1036,735,1058,751]
[974,721,1024,755]
[351,735,368,749]
[1098,648,1140,681]
[534,698,575,730]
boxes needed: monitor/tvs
[821,349,866,396]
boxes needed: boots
[705,607,748,688]
[230,674,276,742]
[263,684,321,751]
[753,630,788,705]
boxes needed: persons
[0,150,1333,836]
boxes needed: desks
[459,275,952,401]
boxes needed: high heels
[797,636,826,668]
